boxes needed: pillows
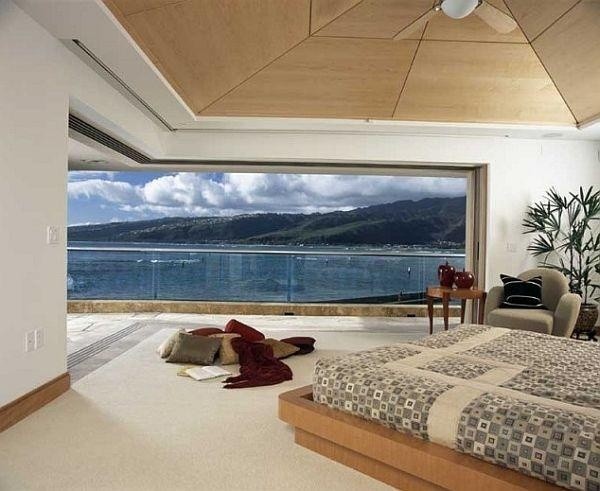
[159,320,315,366]
[498,273,545,310]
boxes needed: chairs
[485,266,582,339]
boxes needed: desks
[425,286,487,334]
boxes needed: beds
[280,323,599,491]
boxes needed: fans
[394,1,520,42]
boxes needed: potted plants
[521,187,600,340]
